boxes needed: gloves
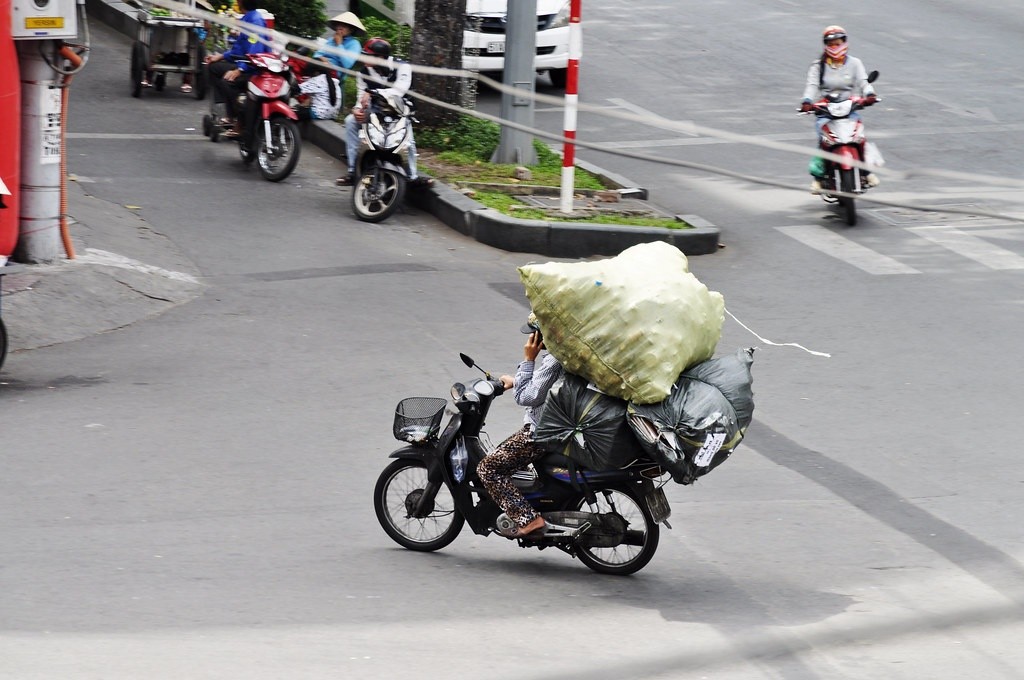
[802,102,812,111]
[863,95,876,106]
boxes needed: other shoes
[140,79,152,87]
[811,180,823,195]
[180,83,192,92]
[219,123,241,137]
[214,115,237,126]
[866,174,880,186]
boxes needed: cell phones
[532,331,543,346]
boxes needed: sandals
[336,173,355,185]
[407,176,434,190]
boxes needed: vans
[357,1,584,94]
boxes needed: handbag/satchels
[450,436,468,483]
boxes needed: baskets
[393,397,448,446]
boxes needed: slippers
[502,527,544,541]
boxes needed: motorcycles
[201,46,311,183]
[336,87,425,223]
[795,70,882,226]
[372,351,673,577]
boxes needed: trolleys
[127,5,208,102]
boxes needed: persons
[801,25,878,194]
[202,0,271,137]
[312,11,366,81]
[299,57,342,119]
[475,313,563,538]
[335,37,434,186]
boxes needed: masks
[825,42,847,59]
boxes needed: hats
[520,312,540,334]
[327,12,366,35]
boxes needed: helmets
[824,25,847,44]
[361,38,393,58]
